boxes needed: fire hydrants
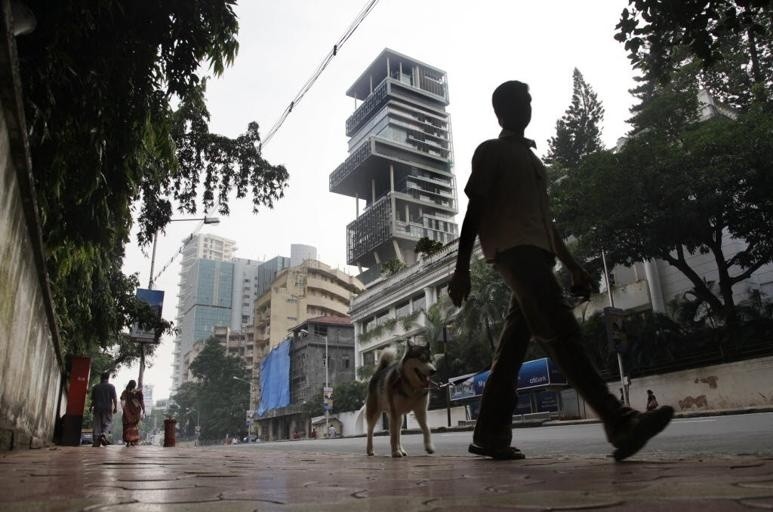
[163,414,177,447]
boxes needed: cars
[81,433,93,445]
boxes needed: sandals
[468,444,525,459]
[605,405,672,460]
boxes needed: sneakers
[92,436,134,448]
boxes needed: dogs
[365,338,437,458]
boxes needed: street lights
[550,170,633,410]
[232,376,253,443]
[300,329,331,437]
[137,217,220,390]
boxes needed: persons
[121,380,145,446]
[449,80,674,460]
[646,389,657,412]
[91,373,117,446]
[295,425,337,440]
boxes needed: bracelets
[454,268,472,274]
